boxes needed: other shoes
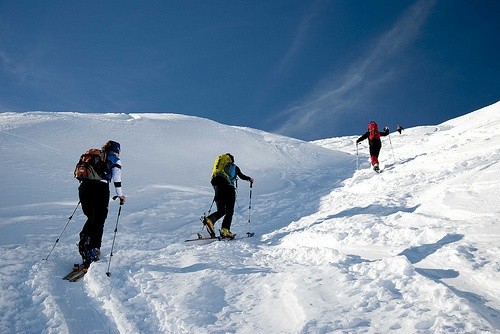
[79,238,89,256]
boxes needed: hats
[104,140,120,154]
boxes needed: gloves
[249,178,254,183]
[120,195,126,203]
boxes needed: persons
[78,140,125,265]
[396,123,404,134]
[356,121,390,171]
[204,153,254,238]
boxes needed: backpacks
[211,154,236,186]
[369,124,380,140]
[74,149,116,181]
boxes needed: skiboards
[184,231,255,247]
[62,260,94,283]
[372,163,385,174]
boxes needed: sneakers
[220,228,233,238]
[202,218,213,233]
